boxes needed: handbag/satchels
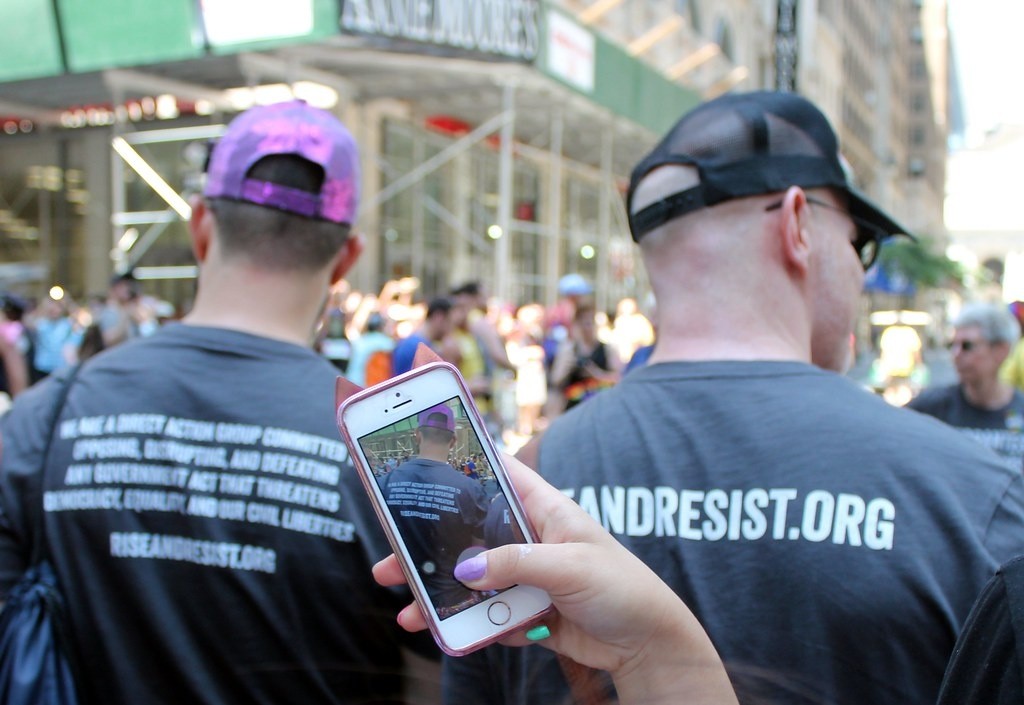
[465,464,471,475]
[0,362,82,705]
[366,351,393,389]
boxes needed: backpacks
[80,309,109,360]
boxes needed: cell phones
[337,360,557,656]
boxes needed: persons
[373,452,740,705]
[882,321,923,390]
[0,271,158,399]
[484,493,515,550]
[433,89,1024,705]
[0,96,415,705]
[905,304,1023,431]
[320,274,656,447]
[362,403,497,621]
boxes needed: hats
[205,98,362,226]
[1007,301,1024,334]
[625,89,917,243]
[418,404,455,433]
[559,275,594,295]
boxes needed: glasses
[946,340,973,351]
[765,196,886,270]
